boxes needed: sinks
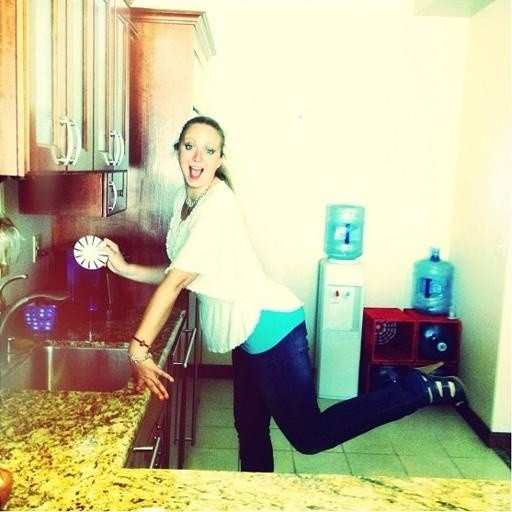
[0,345,137,392]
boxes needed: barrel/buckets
[412,248,453,315]
[371,365,402,388]
[422,324,456,363]
[323,204,363,261]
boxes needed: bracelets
[126,352,152,365]
[132,335,152,349]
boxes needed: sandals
[412,368,467,406]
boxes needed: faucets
[0,274,28,295]
[0,293,70,345]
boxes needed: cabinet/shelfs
[17,171,131,218]
[361,308,462,390]
[166,308,198,470]
[0,0,85,177]
[125,366,170,469]
[85,1,134,172]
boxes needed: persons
[95,117,470,472]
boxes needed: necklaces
[185,185,209,211]
